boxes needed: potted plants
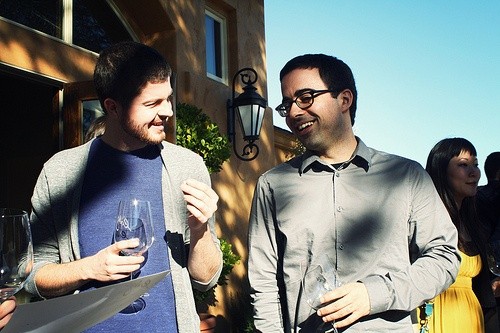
[176,103,242,333]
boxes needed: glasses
[275,89,333,117]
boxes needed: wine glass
[301,255,342,333]
[113,200,155,315]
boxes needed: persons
[247,53,461,333]
[0,40,224,333]
[409,138,500,333]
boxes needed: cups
[487,252,500,276]
[0,210,34,300]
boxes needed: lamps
[226,67,267,162]
